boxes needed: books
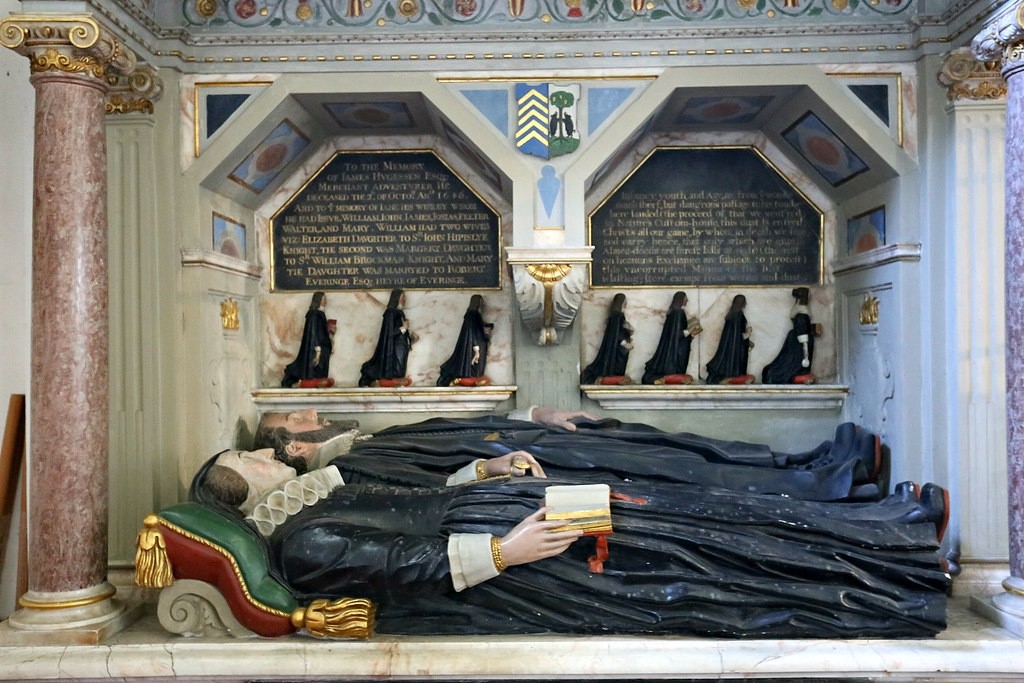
[687,317,703,337]
[544,484,614,536]
[327,319,337,335]
[812,323,823,337]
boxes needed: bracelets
[476,461,490,480]
[492,537,507,571]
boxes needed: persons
[187,450,953,640]
[281,292,337,388]
[580,292,635,385]
[253,405,882,502]
[356,288,420,388]
[641,290,703,385]
[435,294,494,388]
[705,295,755,386]
[762,287,823,384]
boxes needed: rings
[510,467,513,475]
